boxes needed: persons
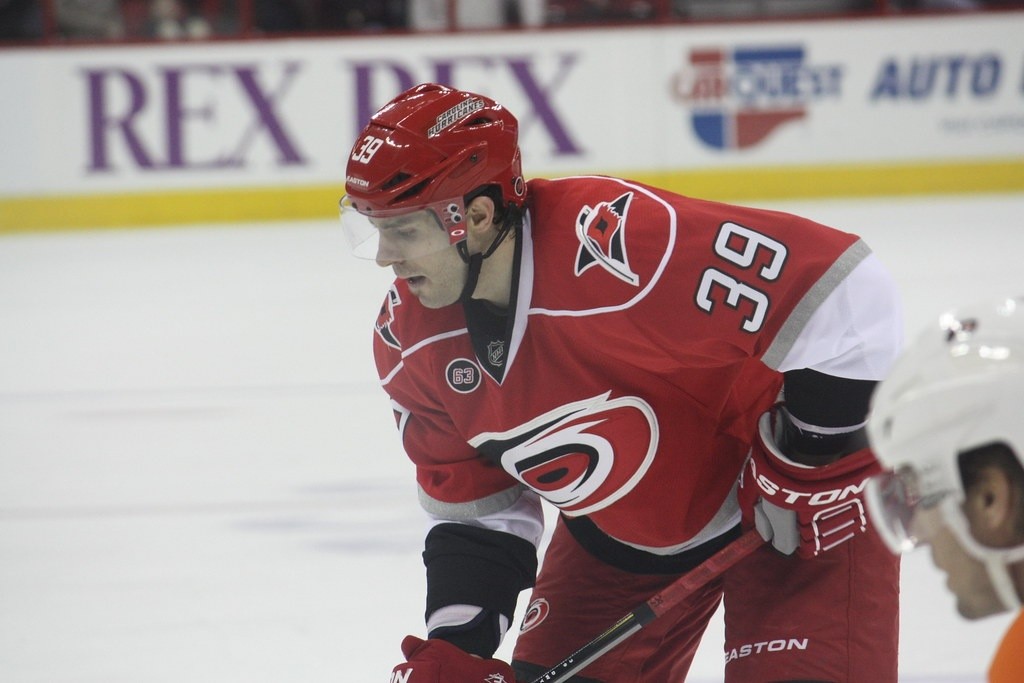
[865,296,1024,683]
[343,83,906,683]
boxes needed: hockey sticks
[529,528,764,683]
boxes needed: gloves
[736,407,888,560]
[390,634,516,683]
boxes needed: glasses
[861,461,993,557]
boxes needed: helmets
[862,298,1024,502]
[345,83,527,219]
